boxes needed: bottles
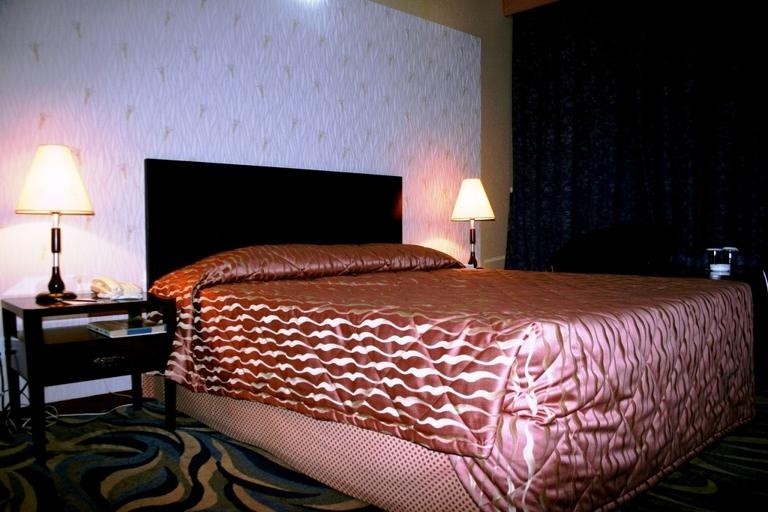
[705,248,722,271]
[722,247,738,271]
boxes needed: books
[87,315,169,339]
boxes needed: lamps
[450,178,496,266]
[15,145,95,305]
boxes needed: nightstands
[1,290,177,460]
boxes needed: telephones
[91,278,143,301]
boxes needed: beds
[144,157,755,512]
